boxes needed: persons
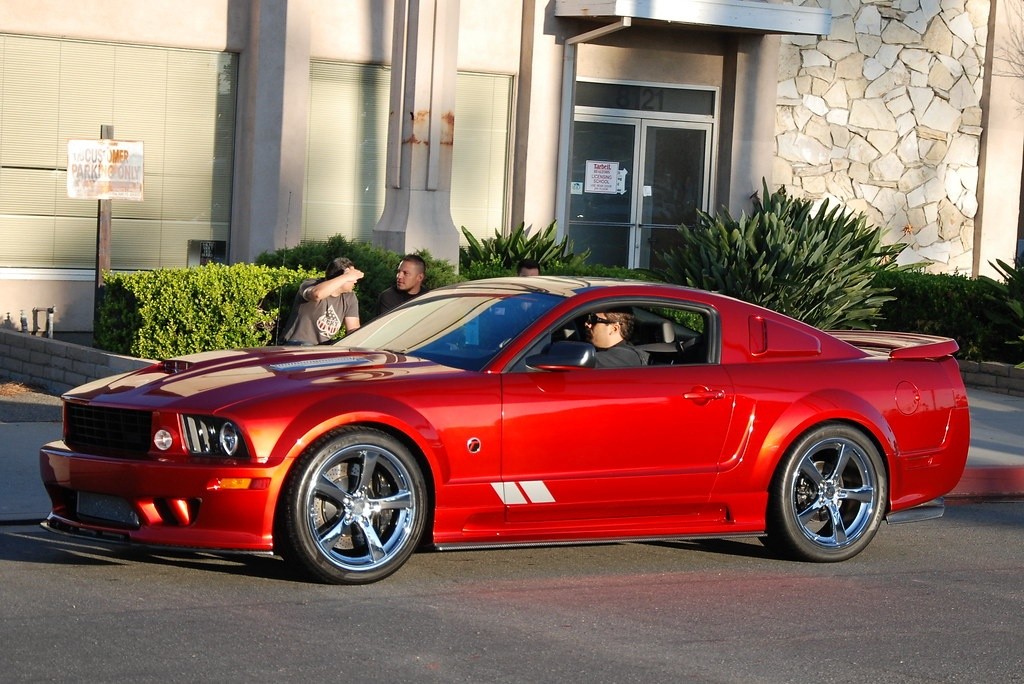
[584,305,647,368]
[375,254,430,317]
[280,257,365,347]
[516,259,540,277]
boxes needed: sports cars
[37,274,972,587]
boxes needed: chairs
[629,319,675,369]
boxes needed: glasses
[585,311,623,326]
